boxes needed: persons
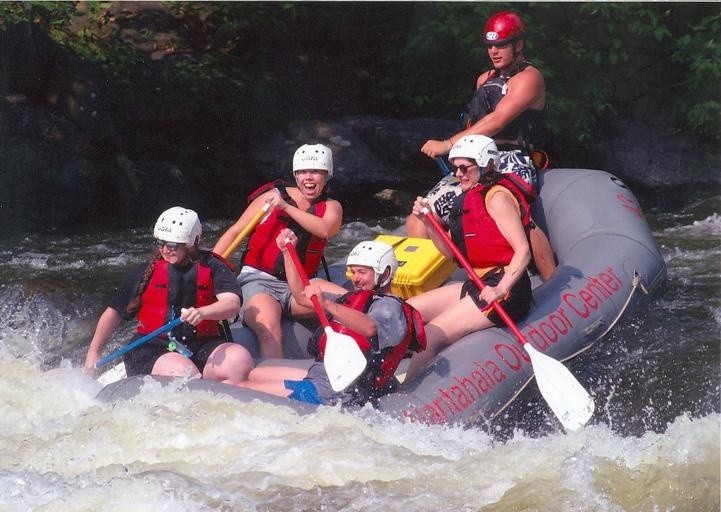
[394,132,533,392]
[418,8,559,287]
[82,205,253,389]
[206,142,350,364]
[238,226,426,408]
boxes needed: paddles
[284,234,368,394]
[420,206,597,433]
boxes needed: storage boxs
[344,234,456,299]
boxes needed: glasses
[450,163,477,174]
[484,41,509,49]
[155,238,180,252]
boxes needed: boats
[93,167,666,442]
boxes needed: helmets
[152,205,204,248]
[345,239,400,288]
[480,9,529,46]
[447,133,500,172]
[291,142,335,181]
